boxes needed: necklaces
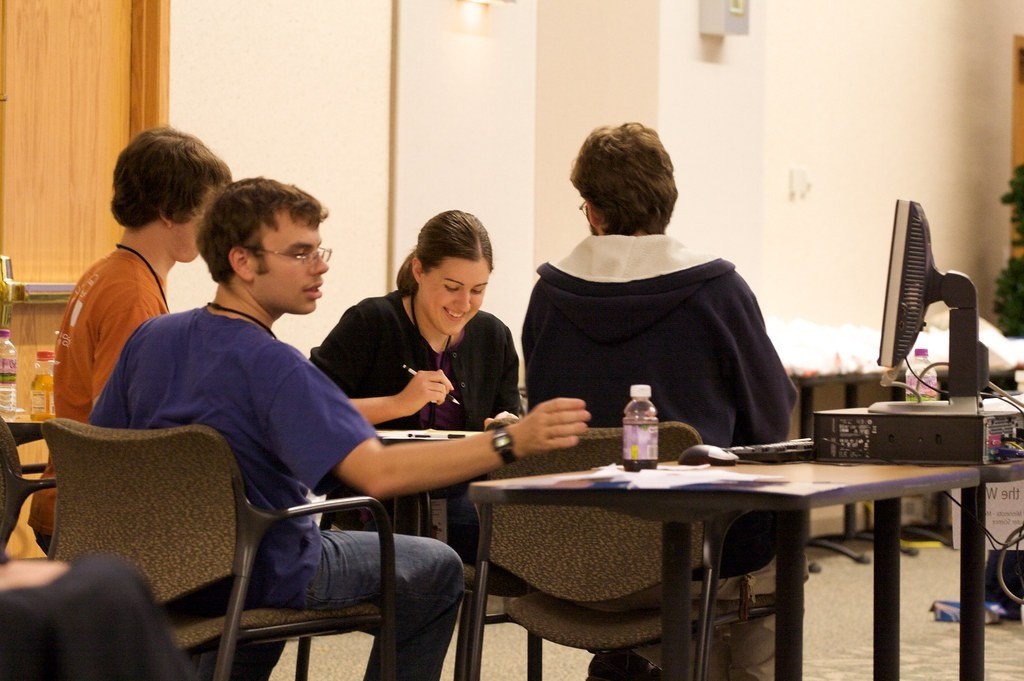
[116,244,170,313]
[208,302,277,339]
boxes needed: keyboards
[725,438,816,462]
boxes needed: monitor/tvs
[867,199,989,416]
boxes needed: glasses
[579,201,588,216]
[242,246,332,267]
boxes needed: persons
[308,210,521,569]
[521,122,799,681]
[88,176,591,681]
[26,125,232,558]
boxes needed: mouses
[677,445,739,467]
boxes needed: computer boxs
[812,397,1023,466]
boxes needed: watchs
[493,426,516,464]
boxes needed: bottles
[0,329,17,422]
[905,349,938,402]
[622,385,658,472]
[30,351,56,422]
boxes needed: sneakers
[585,652,664,681]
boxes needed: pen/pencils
[402,363,460,405]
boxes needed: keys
[746,575,756,603]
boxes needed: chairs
[35,414,403,681]
[292,464,542,681]
[464,418,778,680]
[0,415,58,563]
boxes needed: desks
[376,370,1024,681]
